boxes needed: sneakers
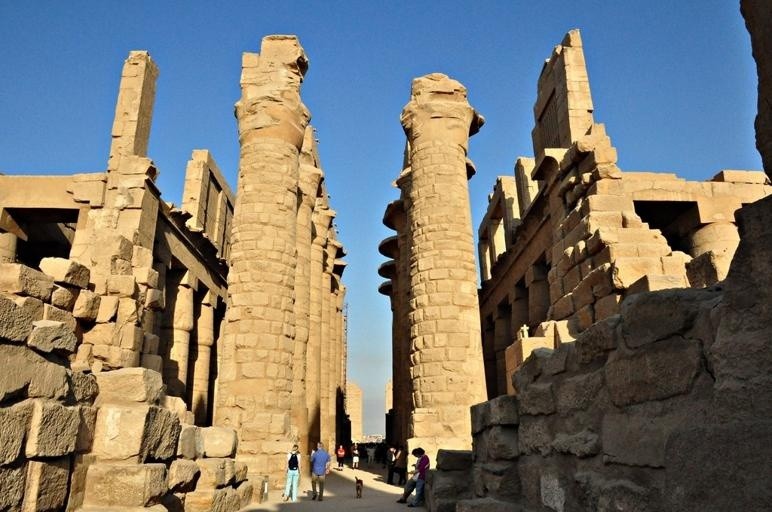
[408,504,418,508]
[283,495,289,501]
[311,493,317,500]
[318,494,323,501]
[396,499,408,504]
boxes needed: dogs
[355,476,363,499]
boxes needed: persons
[367,446,376,465]
[310,442,331,501]
[395,445,407,486]
[350,442,360,470]
[283,445,301,503]
[385,448,396,485]
[337,445,345,471]
[396,448,422,504]
[407,447,430,508]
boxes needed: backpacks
[288,453,298,470]
